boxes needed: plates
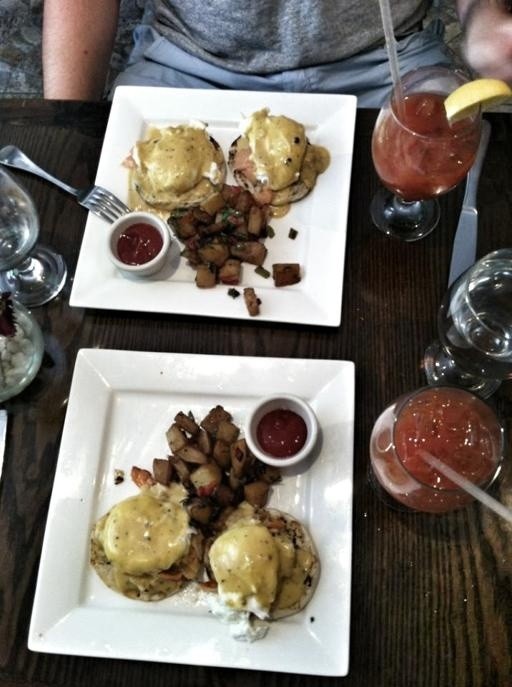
[24,347,359,678]
[67,82,360,329]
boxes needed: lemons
[444,79,512,118]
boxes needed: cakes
[90,496,203,601]
[228,110,317,205]
[121,127,226,211]
[204,508,319,619]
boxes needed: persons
[37,0,512,118]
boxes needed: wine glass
[0,163,70,310]
[368,64,486,244]
[423,246,512,403]
[366,382,508,516]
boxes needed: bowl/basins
[105,209,173,278]
[243,393,320,469]
[0,298,46,402]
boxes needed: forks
[0,144,130,226]
